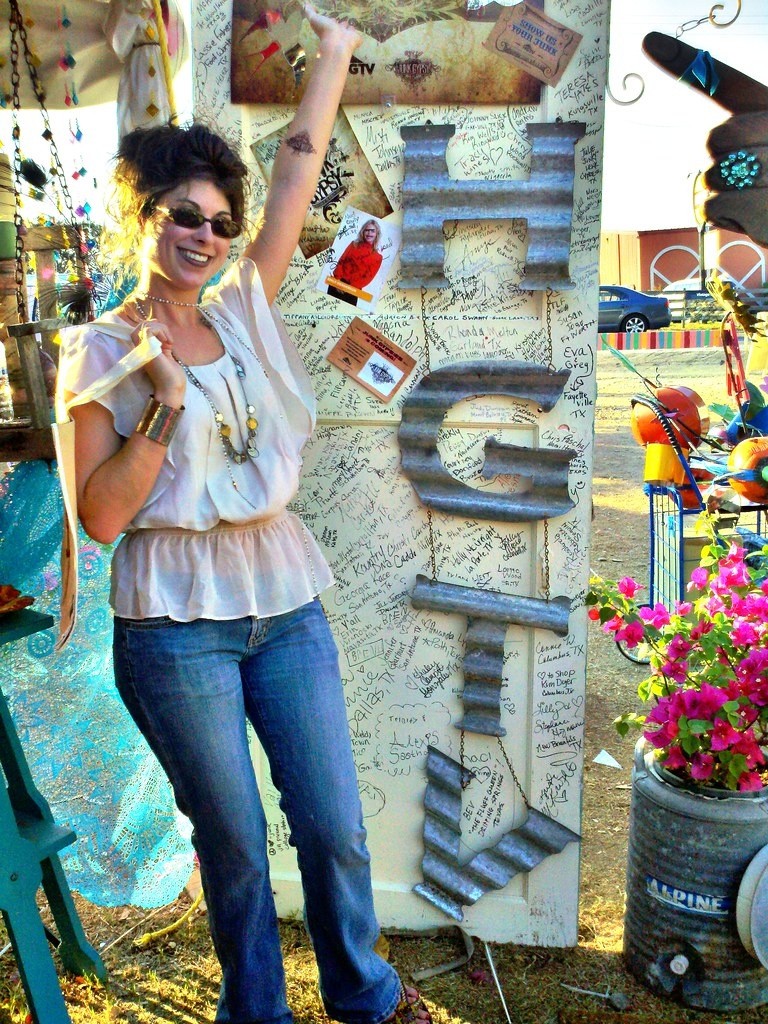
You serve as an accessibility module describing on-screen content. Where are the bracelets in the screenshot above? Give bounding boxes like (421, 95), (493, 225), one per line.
(134, 394), (186, 447)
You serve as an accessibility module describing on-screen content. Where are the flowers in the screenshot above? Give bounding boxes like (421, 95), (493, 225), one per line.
(588, 501), (768, 793)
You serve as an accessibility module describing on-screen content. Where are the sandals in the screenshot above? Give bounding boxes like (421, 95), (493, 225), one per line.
(380, 981), (433, 1024)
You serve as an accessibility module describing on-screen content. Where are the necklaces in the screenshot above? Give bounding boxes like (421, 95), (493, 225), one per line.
(132, 286), (267, 509)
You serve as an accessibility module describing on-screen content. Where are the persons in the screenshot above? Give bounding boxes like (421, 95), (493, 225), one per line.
(327, 219), (383, 306)
(54, 3), (434, 1024)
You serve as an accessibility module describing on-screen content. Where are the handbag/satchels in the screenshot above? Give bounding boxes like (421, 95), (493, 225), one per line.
(50, 323), (161, 652)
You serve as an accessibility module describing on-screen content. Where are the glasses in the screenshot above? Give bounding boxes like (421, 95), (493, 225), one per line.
(150, 204), (242, 239)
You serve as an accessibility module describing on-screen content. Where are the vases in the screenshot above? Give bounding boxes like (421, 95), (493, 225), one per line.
(622, 736), (768, 1013)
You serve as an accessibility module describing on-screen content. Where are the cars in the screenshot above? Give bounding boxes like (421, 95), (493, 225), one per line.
(657, 276), (736, 323)
(597, 285), (672, 333)
